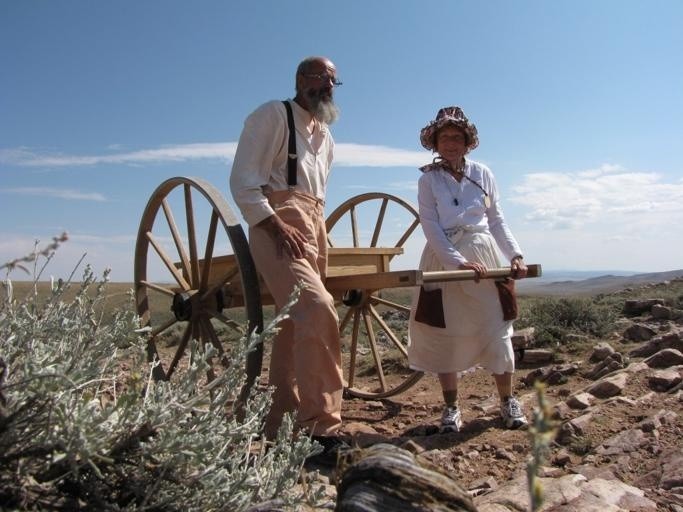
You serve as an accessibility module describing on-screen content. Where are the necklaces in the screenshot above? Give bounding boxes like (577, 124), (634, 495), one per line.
(438, 162), (464, 208)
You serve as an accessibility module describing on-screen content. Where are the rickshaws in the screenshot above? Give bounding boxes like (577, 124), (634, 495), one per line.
(132, 173), (542, 426)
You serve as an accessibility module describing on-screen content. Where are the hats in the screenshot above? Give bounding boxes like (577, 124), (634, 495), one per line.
(420, 106), (480, 154)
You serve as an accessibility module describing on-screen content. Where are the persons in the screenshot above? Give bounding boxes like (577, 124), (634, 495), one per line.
(406, 107), (530, 429)
(227, 58), (347, 486)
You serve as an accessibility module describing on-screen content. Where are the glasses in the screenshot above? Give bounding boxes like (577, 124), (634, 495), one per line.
(305, 73), (340, 88)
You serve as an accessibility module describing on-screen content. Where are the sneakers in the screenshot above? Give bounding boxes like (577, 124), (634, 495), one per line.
(499, 397), (528, 429)
(296, 432), (354, 466)
(440, 405), (462, 434)
